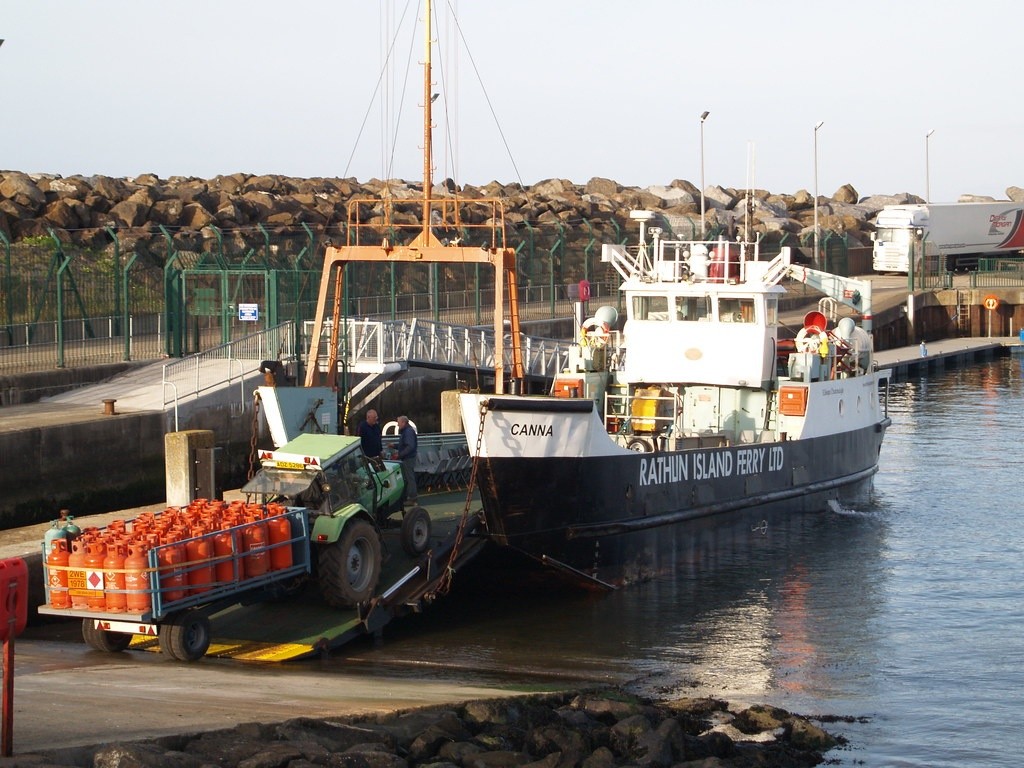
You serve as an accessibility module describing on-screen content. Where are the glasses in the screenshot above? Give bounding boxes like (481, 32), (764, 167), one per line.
(369, 416), (378, 420)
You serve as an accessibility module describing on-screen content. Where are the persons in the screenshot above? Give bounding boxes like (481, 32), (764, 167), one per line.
(388, 416), (418, 506)
(357, 409), (384, 460)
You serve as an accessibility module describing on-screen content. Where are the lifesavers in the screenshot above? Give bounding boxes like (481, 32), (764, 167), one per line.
(794, 325), (823, 354)
(627, 439), (649, 453)
(580, 318), (610, 348)
(381, 419), (418, 435)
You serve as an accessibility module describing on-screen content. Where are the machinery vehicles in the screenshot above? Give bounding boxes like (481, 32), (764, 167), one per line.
(34, 431), (432, 664)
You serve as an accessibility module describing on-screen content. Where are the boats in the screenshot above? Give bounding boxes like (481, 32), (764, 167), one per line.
(252, 210), (893, 588)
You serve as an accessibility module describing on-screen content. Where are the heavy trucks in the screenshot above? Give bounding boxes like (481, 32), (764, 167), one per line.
(870, 201), (1024, 276)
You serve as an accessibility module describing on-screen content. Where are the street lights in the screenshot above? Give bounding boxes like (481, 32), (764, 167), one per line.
(700, 110), (712, 242)
(813, 121), (824, 269)
(925, 129), (936, 203)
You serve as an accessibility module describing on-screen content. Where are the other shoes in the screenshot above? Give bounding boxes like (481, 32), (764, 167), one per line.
(403, 499), (418, 506)
